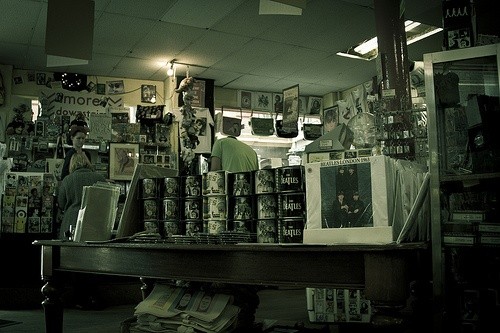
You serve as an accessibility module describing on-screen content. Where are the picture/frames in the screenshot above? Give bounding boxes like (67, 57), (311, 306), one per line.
(141, 84), (157, 103)
(322, 105), (339, 137)
(109, 142), (141, 181)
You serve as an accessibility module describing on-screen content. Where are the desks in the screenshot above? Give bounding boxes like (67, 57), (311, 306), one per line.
(32, 238), (430, 333)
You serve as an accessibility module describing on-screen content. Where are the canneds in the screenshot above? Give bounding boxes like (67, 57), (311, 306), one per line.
(141, 165), (307, 244)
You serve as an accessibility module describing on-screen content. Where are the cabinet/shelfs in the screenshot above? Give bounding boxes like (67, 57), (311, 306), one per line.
(423, 41), (500, 333)
(139, 117), (176, 170)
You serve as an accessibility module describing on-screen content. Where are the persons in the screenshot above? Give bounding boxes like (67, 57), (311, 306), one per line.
(211, 135), (259, 172)
(61, 125), (92, 180)
(59, 152), (107, 238)
(334, 165), (365, 227)
